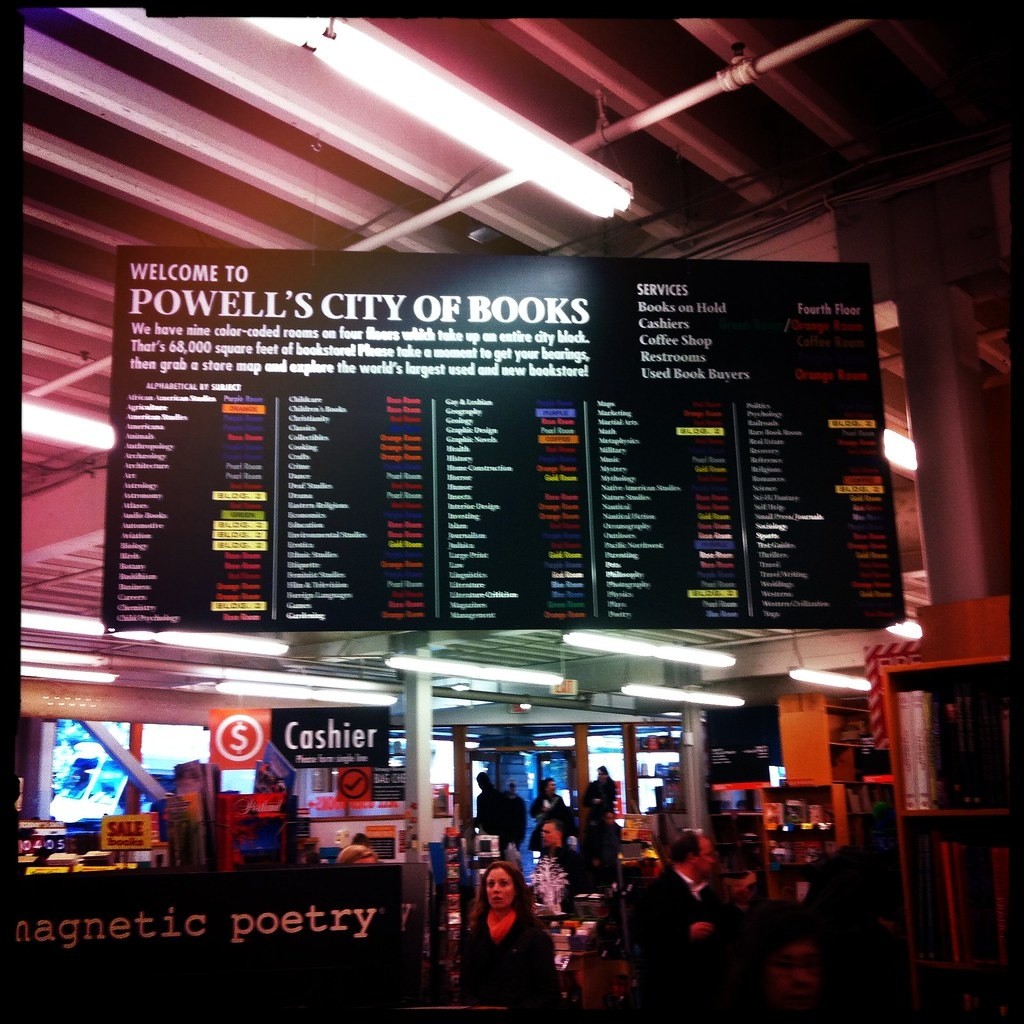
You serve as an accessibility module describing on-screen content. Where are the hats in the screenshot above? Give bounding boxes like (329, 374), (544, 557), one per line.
(337, 845), (372, 864)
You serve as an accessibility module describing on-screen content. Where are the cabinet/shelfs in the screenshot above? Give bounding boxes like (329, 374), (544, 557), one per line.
(759, 782), (849, 902)
(827, 703), (893, 847)
(713, 812), (765, 876)
(633, 721), (688, 814)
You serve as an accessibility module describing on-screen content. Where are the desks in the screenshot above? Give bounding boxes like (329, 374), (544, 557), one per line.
(555, 951), (628, 1008)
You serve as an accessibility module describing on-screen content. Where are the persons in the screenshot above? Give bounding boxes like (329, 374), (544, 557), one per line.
(459, 861), (564, 1004)
(626, 830), (822, 1024)
(476, 766), (620, 896)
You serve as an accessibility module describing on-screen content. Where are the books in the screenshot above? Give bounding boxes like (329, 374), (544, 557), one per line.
(894, 692), (1024, 963)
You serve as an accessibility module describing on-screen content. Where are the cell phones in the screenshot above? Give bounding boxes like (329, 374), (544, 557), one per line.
(49, 749), (129, 823)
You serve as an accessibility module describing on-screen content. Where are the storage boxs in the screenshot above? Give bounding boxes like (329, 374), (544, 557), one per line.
(841, 730), (859, 740)
(660, 736), (670, 750)
(804, 841), (823, 862)
(640, 737), (649, 750)
(825, 841), (835, 853)
(786, 800), (807, 823)
(797, 881), (814, 902)
(648, 735), (659, 750)
(470, 833), (609, 952)
(794, 841), (805, 864)
(764, 802), (785, 824)
(807, 805), (831, 822)
(769, 840), (795, 863)
(848, 720), (867, 735)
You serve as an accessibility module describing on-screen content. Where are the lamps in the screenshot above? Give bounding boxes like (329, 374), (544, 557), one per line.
(620, 657), (746, 709)
(19, 607), (398, 706)
(786, 629), (874, 693)
(382, 630), (566, 685)
(563, 628), (737, 668)
(255, 15), (636, 220)
